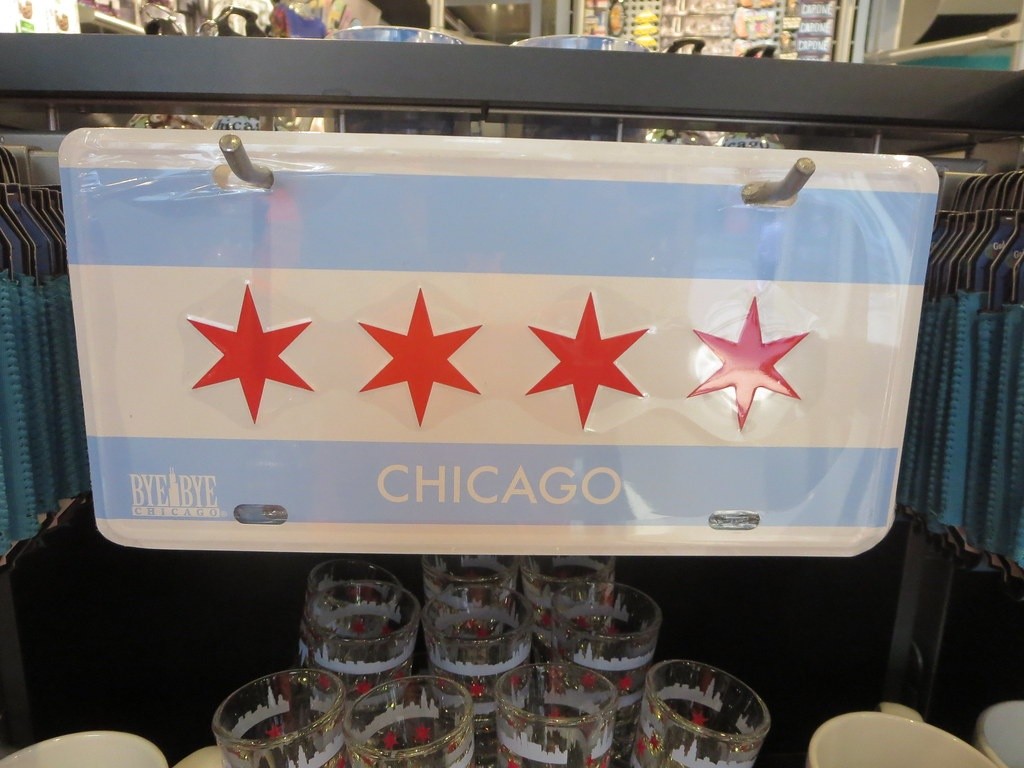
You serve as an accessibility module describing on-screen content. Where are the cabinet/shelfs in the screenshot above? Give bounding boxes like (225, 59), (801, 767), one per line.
(572, 0), (856, 63)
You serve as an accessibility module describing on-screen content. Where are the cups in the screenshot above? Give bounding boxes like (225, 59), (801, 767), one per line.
(210, 556), (772, 768)
(971, 699), (1024, 768)
(804, 702), (996, 768)
(509, 35), (649, 143)
(0, 730), (169, 768)
(323, 25), (473, 137)
